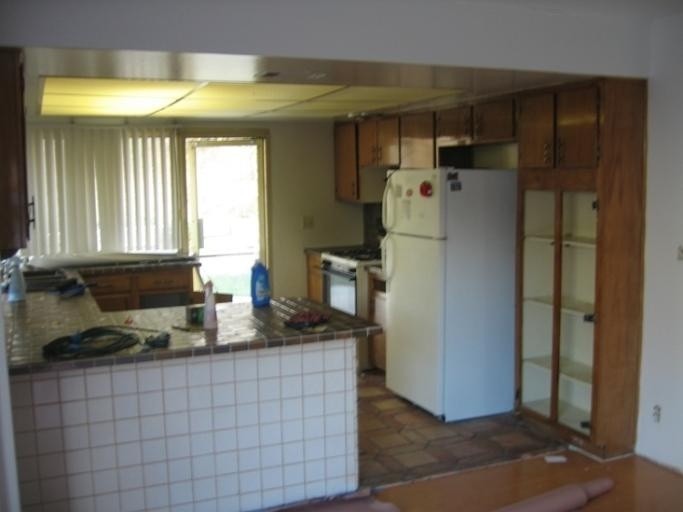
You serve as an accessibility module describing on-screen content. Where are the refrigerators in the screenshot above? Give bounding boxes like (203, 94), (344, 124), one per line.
(380, 166), (522, 426)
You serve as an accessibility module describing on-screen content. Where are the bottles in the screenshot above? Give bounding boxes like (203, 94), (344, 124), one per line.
(6, 263), (27, 302)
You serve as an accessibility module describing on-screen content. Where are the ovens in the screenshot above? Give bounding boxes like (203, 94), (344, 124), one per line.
(317, 268), (370, 373)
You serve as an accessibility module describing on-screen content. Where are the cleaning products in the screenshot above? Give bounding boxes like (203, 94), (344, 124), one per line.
(6, 257), (27, 302)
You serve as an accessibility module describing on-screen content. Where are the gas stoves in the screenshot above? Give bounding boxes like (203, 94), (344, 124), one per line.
(320, 243), (381, 271)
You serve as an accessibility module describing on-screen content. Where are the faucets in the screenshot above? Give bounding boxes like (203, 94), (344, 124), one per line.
(2, 258), (29, 283)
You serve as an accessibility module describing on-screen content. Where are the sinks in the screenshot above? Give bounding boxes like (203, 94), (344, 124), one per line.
(8, 268), (65, 279)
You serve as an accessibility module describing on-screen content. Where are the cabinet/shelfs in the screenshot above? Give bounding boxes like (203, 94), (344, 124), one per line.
(370, 278), (385, 372)
(306, 253), (322, 302)
(433, 96), (515, 145)
(0, 47), (36, 261)
(84, 267), (232, 311)
(356, 117), (399, 202)
(332, 121), (358, 202)
(399, 112), (434, 168)
